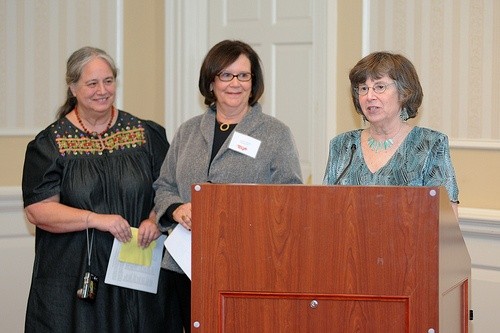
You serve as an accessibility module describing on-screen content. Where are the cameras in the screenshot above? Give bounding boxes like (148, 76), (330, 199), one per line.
(77, 272), (99, 303)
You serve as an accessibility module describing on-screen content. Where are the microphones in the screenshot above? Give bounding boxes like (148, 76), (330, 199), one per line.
(334, 144), (356, 185)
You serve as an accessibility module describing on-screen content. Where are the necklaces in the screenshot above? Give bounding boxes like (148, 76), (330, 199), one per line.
(365, 123), (407, 153)
(213, 106), (251, 131)
(73, 103), (115, 137)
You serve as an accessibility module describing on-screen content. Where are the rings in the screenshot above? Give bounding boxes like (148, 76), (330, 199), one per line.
(181, 214), (187, 220)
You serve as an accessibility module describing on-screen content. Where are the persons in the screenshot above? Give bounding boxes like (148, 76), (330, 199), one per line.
(322, 51), (459, 223)
(151, 39), (304, 333)
(21, 45), (171, 333)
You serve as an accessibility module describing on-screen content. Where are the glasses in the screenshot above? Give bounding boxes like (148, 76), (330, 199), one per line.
(214, 71), (254, 82)
(354, 81), (397, 96)
